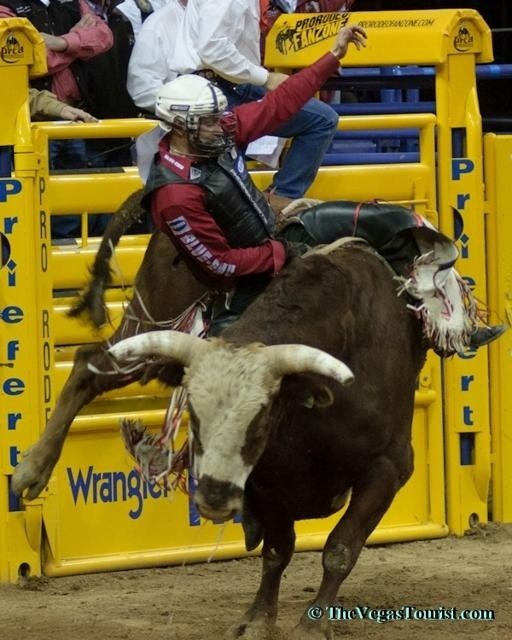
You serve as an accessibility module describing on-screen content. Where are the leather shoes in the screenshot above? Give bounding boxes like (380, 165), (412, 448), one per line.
(429, 319), (510, 360)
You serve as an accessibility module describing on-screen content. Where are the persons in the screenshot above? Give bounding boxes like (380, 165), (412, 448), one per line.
(260, 0), (350, 102)
(168, 1), (339, 209)
(0, 0), (192, 263)
(121, 22), (509, 483)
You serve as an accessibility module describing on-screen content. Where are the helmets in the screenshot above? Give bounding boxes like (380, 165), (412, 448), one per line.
(153, 72), (229, 135)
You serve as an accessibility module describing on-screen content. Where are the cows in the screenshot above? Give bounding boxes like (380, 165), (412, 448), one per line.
(8, 177), (429, 639)
